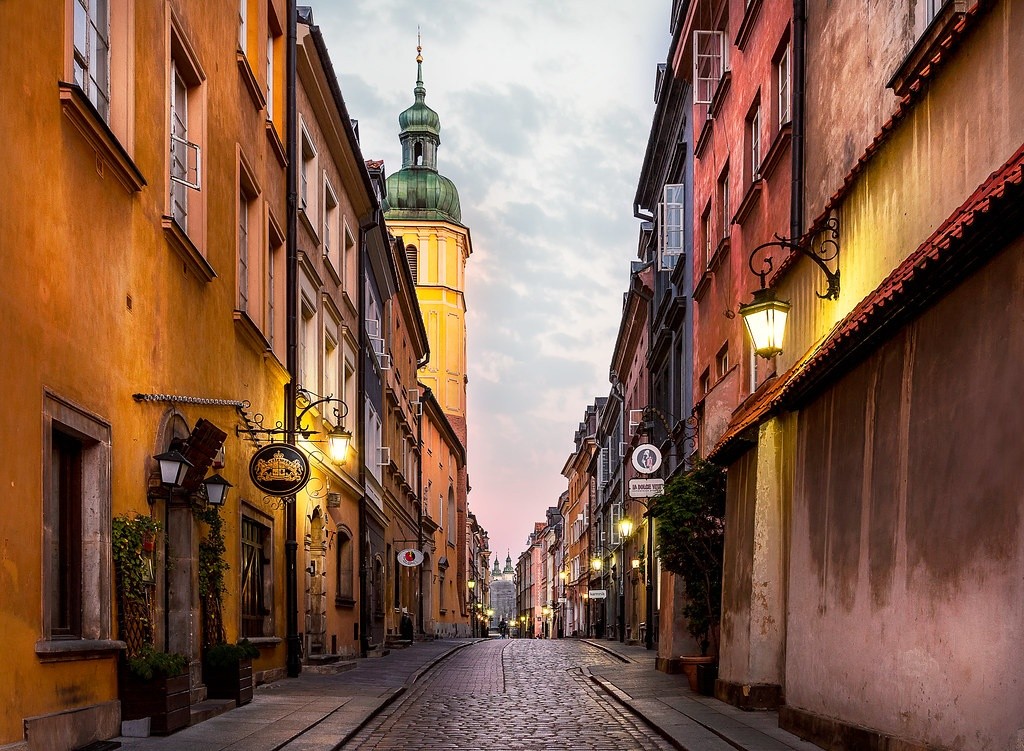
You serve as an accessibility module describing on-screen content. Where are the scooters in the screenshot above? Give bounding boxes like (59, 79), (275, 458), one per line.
(498, 623), (507, 638)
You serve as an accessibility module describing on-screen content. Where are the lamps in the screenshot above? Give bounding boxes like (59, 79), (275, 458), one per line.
(631, 559), (643, 570)
(593, 546), (612, 572)
(235, 383), (353, 467)
(616, 498), (650, 538)
(148, 447), (196, 506)
(200, 474), (233, 518)
(738, 201), (840, 360)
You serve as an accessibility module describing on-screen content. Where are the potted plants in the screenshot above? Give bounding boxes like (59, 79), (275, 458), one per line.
(202, 639), (260, 706)
(653, 461), (727, 691)
(126, 644), (192, 735)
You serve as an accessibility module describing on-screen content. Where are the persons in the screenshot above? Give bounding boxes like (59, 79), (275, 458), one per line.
(498, 618), (506, 638)
